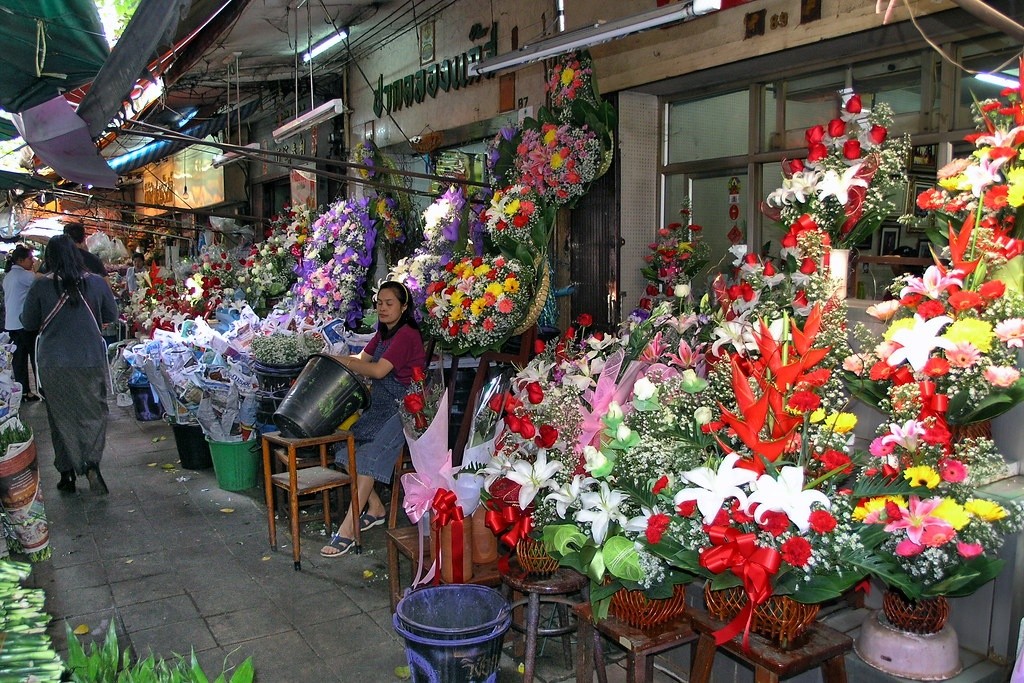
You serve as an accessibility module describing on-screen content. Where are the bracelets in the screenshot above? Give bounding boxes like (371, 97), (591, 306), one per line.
(347, 359), (355, 366)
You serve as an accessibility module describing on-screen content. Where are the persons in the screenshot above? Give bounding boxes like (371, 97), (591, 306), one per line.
(125, 254), (148, 293)
(65, 224), (111, 330)
(320, 282), (424, 558)
(3, 244), (43, 401)
(19, 236), (118, 496)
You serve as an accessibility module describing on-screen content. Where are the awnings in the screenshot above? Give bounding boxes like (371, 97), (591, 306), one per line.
(0, 2), (493, 198)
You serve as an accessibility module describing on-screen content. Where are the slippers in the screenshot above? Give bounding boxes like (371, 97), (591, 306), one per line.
(359, 510), (386, 531)
(320, 531), (357, 557)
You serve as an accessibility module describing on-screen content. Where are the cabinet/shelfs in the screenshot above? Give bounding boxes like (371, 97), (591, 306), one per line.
(262, 430), (363, 570)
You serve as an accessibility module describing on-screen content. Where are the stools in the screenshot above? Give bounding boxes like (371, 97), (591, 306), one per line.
(385, 438), (415, 529)
(690, 609), (853, 683)
(415, 553), (505, 589)
(573, 600), (698, 683)
(496, 556), (591, 683)
(387, 527), (430, 615)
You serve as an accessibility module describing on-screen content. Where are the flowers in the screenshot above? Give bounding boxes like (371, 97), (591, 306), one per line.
(236, 56), (1024, 597)
(136, 249), (232, 331)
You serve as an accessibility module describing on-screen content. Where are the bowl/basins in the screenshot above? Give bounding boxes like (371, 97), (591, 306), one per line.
(851, 610), (962, 681)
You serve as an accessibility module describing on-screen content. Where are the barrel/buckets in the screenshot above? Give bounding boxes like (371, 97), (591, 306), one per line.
(204, 432), (259, 491)
(129, 377), (165, 421)
(272, 353), (372, 438)
(392, 584), (512, 683)
(169, 421), (212, 469)
(249, 389), (290, 478)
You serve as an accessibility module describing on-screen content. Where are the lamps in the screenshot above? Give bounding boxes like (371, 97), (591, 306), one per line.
(273, 0), (343, 145)
(302, 26), (350, 64)
(214, 53), (259, 166)
(477, 0), (721, 79)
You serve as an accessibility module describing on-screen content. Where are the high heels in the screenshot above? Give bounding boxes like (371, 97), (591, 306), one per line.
(57, 469), (76, 495)
(21, 393), (40, 402)
(84, 463), (109, 495)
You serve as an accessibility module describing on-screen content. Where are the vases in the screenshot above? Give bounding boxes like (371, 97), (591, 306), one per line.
(706, 575), (821, 643)
(583, 118), (614, 179)
(514, 520), (562, 576)
(511, 251), (551, 338)
(882, 587), (951, 634)
(594, 577), (687, 631)
(825, 248), (853, 301)
(947, 417), (994, 457)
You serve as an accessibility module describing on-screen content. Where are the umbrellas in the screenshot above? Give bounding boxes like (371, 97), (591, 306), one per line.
(12, 95), (123, 194)
